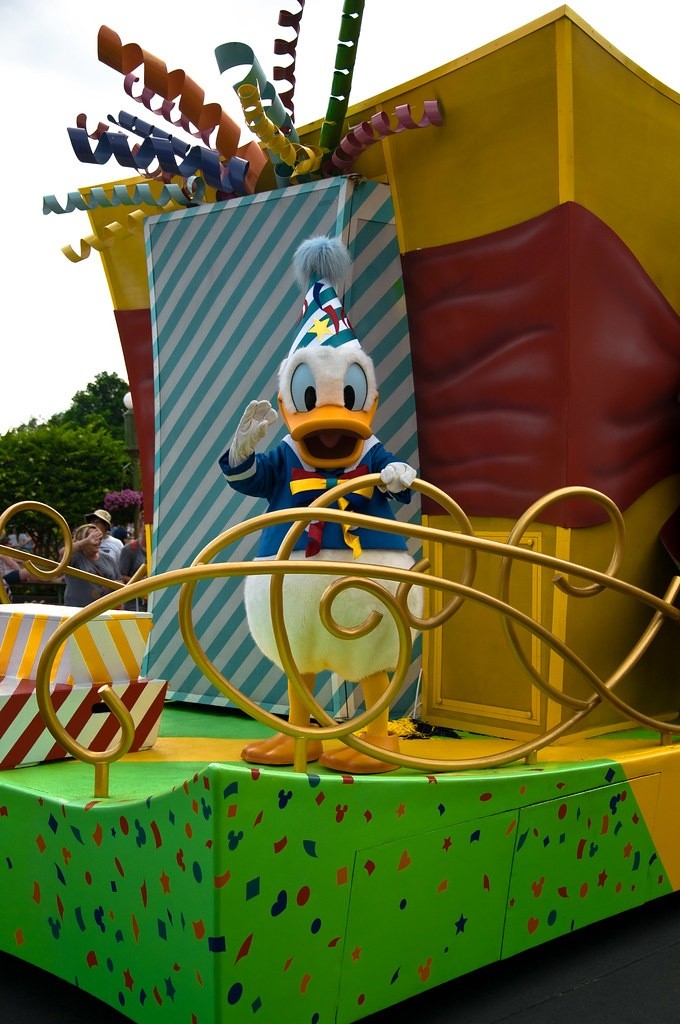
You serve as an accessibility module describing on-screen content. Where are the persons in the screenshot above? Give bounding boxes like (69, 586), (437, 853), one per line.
(120, 510), (147, 612)
(58, 524), (122, 608)
(9, 527), (36, 553)
(84, 508), (124, 567)
(0, 538), (48, 584)
(109, 520), (135, 545)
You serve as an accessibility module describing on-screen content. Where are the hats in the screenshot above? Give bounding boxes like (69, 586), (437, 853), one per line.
(84, 509), (112, 532)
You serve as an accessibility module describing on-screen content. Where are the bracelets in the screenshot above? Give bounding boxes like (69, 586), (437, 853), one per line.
(76, 540), (82, 551)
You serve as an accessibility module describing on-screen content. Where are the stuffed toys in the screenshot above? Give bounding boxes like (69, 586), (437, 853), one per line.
(217, 234), (416, 774)
